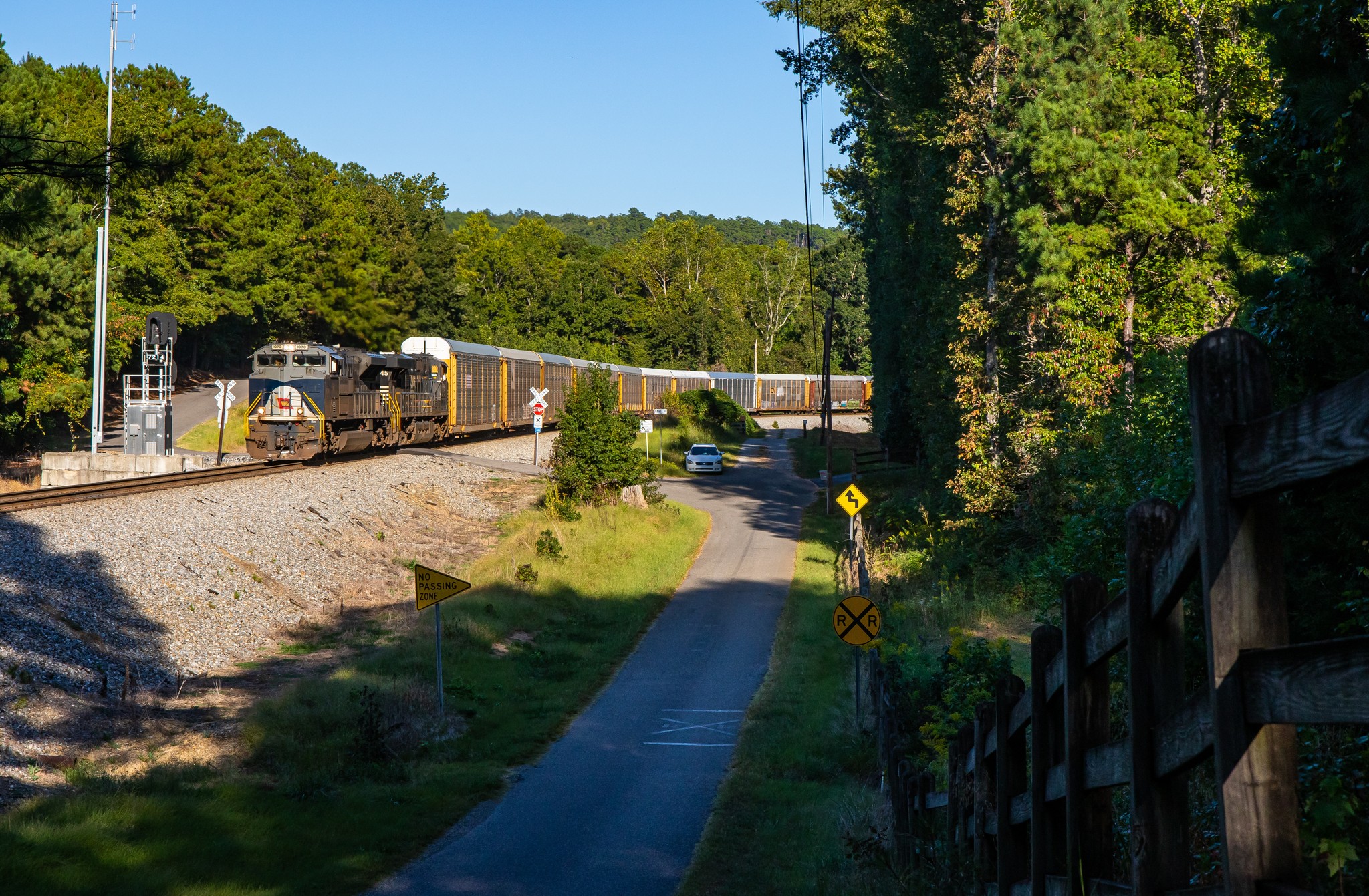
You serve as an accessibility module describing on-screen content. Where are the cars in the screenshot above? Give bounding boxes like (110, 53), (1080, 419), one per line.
(683, 444), (725, 473)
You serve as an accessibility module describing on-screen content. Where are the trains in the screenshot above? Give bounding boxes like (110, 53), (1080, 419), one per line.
(243, 336), (873, 466)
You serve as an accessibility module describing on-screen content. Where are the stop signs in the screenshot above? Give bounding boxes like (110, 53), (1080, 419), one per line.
(533, 403), (545, 416)
